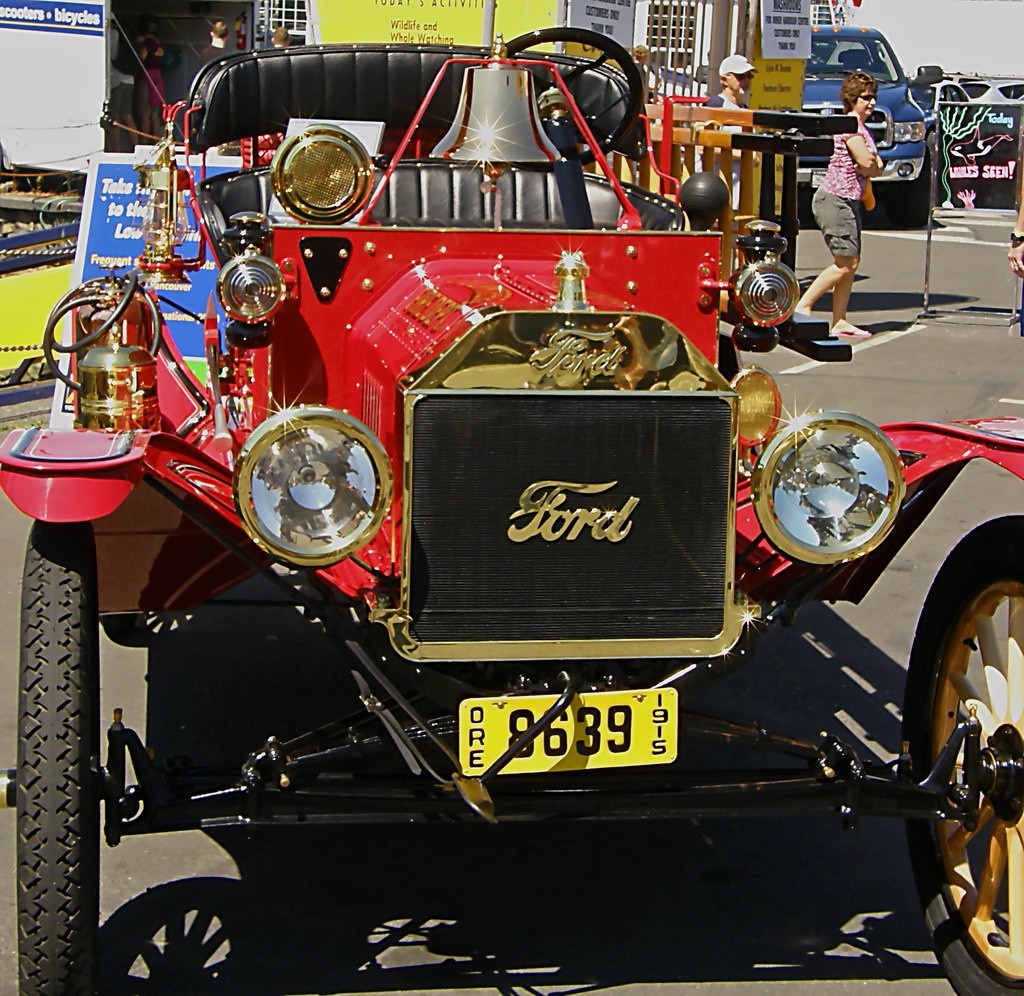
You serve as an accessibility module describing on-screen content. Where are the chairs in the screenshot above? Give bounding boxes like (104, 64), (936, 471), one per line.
(839, 48), (873, 69)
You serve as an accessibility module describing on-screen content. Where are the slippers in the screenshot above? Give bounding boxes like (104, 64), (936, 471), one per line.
(830, 328), (872, 340)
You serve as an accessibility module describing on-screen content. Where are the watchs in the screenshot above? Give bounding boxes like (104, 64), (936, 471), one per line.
(1011, 228), (1024, 243)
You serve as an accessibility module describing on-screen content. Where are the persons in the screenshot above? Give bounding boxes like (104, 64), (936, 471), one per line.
(633, 44), (663, 104)
(109, 22), (165, 151)
(1007, 196), (1024, 336)
(199, 21), (234, 71)
(271, 27), (291, 47)
(796, 72), (883, 339)
(694, 55), (758, 209)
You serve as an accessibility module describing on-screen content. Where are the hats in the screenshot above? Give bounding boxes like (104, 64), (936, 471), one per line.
(718, 55), (758, 77)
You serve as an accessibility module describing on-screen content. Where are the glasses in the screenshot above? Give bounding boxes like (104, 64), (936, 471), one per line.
(859, 94), (878, 100)
(736, 73), (754, 80)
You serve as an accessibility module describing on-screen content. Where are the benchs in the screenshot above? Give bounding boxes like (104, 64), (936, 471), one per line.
(186, 42), (686, 270)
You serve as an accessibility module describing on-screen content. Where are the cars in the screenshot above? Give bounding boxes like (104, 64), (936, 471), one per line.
(0, 30), (1023, 993)
(759, 25), (946, 227)
